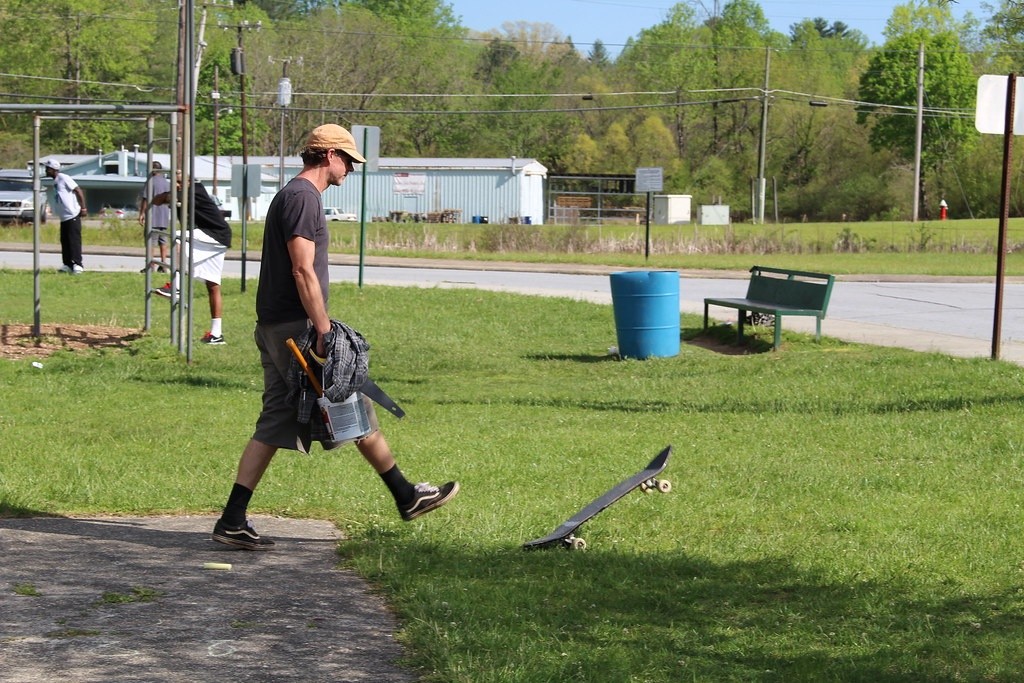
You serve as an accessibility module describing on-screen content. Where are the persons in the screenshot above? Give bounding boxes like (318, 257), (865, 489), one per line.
(139, 161), (170, 273)
(212, 124), (460, 547)
(153, 171), (231, 345)
(43, 159), (87, 272)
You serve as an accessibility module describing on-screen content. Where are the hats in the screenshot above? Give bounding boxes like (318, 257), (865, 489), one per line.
(42, 159), (60, 170)
(305, 124), (367, 163)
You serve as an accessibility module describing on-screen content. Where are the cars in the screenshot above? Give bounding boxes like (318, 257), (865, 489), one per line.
(100, 202), (141, 221)
(323, 207), (358, 223)
(0, 168), (50, 225)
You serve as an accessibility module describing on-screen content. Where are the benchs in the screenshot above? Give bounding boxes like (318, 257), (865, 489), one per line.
(704, 266), (834, 351)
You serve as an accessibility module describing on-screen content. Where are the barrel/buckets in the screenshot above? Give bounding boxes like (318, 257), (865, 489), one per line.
(317, 365), (372, 443)
(608, 270), (680, 360)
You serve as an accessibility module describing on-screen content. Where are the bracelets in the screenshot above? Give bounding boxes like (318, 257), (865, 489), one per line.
(81, 209), (87, 211)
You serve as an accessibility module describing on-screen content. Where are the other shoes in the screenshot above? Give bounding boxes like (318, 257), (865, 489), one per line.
(157, 267), (165, 274)
(140, 268), (146, 273)
(59, 265), (71, 272)
(73, 263), (83, 273)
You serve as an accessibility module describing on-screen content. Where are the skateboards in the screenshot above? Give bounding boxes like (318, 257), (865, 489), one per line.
(524, 444), (673, 549)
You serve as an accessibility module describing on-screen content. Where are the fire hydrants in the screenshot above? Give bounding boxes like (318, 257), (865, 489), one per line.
(939, 199), (949, 220)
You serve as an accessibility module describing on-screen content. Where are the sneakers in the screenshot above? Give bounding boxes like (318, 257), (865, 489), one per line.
(156, 283), (180, 297)
(200, 332), (224, 346)
(213, 519), (275, 550)
(398, 481), (459, 520)
